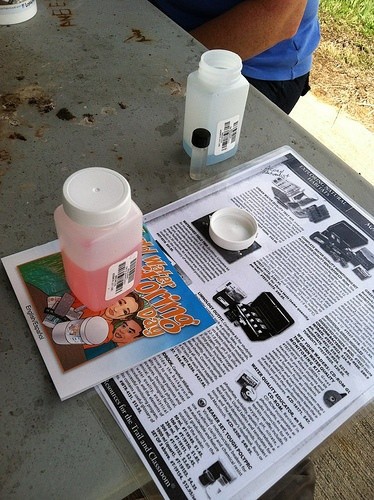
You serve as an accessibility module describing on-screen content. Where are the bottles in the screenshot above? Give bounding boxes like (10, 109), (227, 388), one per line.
(182, 49), (250, 166)
(190, 128), (211, 180)
(53, 166), (144, 311)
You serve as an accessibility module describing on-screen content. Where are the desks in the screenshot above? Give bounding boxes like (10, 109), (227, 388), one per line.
(0, 0), (374, 500)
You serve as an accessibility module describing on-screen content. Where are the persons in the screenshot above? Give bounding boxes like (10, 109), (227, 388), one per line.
(148, 0), (321, 117)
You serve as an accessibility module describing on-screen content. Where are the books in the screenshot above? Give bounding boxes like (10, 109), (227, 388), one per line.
(2, 220), (217, 401)
